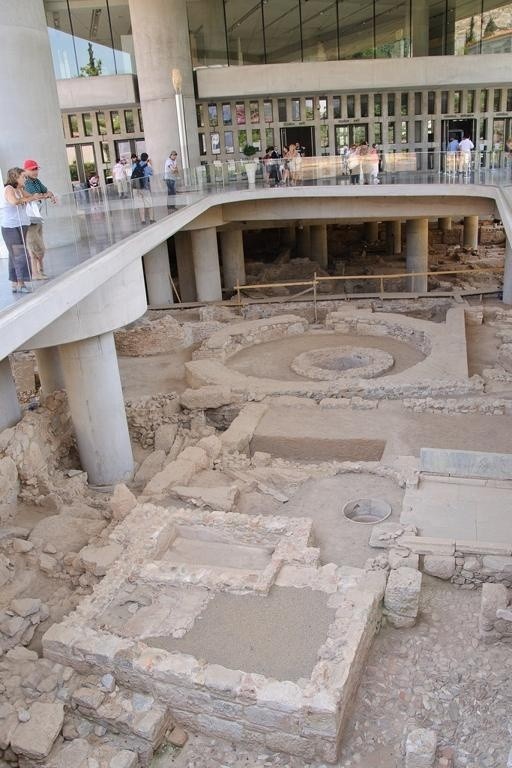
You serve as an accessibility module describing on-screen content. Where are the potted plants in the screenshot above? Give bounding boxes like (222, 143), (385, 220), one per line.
(243, 144), (258, 183)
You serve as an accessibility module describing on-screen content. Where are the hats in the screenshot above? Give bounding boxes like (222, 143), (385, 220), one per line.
(24, 160), (40, 171)
(170, 151), (178, 155)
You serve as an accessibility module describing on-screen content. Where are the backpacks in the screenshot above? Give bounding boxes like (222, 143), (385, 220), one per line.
(132, 162), (148, 189)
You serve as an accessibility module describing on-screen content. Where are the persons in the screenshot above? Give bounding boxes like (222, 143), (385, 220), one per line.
(129, 152), (157, 224)
(89, 175), (100, 193)
(163, 150), (180, 215)
(445, 134), (475, 174)
(261, 141), (306, 184)
(338, 139), (385, 184)
(113, 159), (128, 199)
(131, 154), (141, 171)
(1, 166), (41, 293)
(19, 159), (57, 280)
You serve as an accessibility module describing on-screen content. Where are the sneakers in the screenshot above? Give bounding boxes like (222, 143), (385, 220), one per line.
(10, 286), (29, 293)
(141, 220), (155, 225)
(32, 273), (48, 280)
